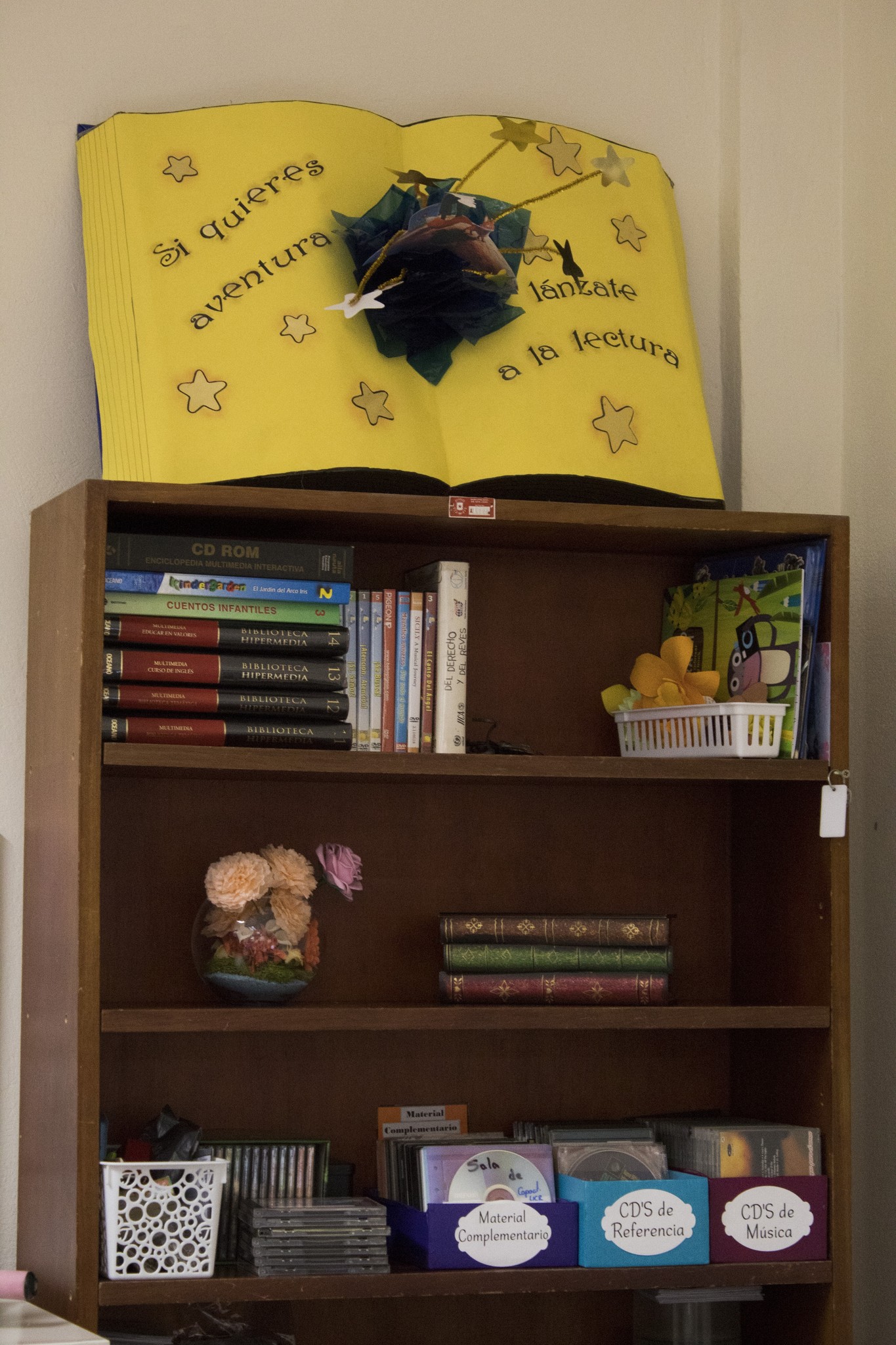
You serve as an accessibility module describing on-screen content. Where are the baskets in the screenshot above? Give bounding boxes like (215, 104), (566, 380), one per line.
(100, 1156), (229, 1278)
(613, 703), (790, 758)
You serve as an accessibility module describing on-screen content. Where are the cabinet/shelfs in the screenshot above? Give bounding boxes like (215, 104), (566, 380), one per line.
(16, 477), (864, 1345)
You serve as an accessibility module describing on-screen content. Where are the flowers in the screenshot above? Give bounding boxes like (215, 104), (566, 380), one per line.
(196, 835), (363, 945)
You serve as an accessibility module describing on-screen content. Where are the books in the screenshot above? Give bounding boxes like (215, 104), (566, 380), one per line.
(426, 902), (680, 1007)
(72, 99), (731, 510)
(99, 518), (847, 761)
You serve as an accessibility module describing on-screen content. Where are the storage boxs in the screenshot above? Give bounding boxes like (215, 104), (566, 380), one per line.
(372, 1197), (577, 1274)
(555, 1168), (710, 1267)
(691, 1168), (828, 1263)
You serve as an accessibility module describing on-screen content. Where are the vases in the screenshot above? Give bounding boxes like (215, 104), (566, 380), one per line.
(192, 894), (321, 1006)
